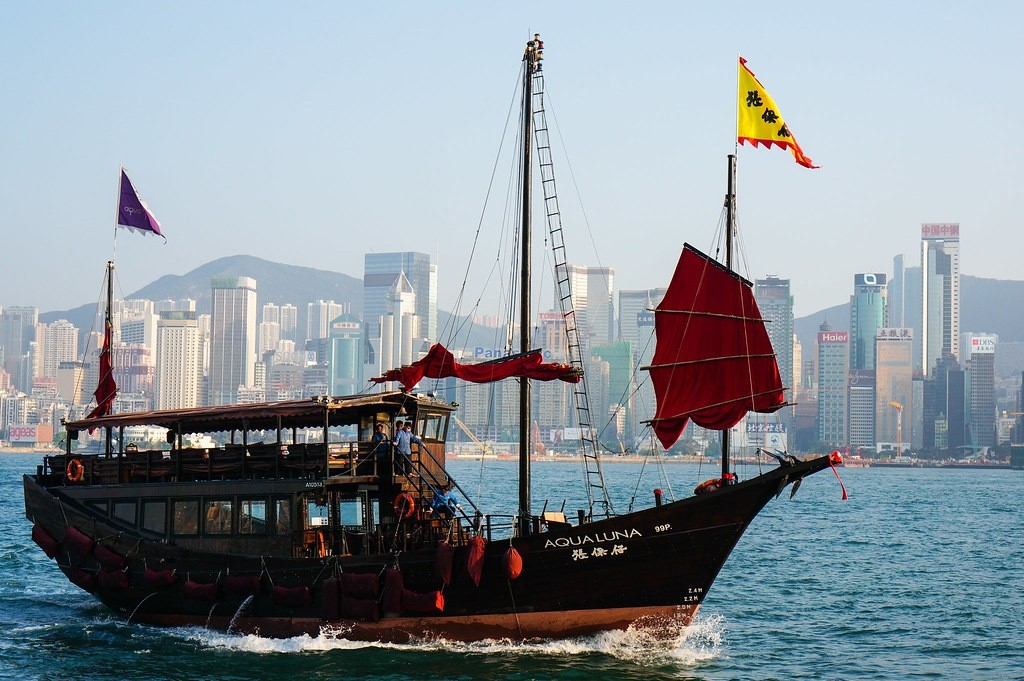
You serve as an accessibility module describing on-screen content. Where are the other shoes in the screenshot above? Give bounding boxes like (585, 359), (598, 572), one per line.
(407, 473), (420, 478)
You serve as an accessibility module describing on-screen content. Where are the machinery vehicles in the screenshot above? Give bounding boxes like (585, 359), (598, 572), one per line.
(887, 400), (903, 463)
(1002, 410), (1024, 418)
(452, 414), (497, 456)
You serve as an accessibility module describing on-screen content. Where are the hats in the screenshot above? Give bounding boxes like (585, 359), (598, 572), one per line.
(442, 484), (451, 491)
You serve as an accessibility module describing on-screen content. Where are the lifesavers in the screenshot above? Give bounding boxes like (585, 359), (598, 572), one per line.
(394, 493), (415, 519)
(694, 478), (721, 494)
(67, 459), (82, 482)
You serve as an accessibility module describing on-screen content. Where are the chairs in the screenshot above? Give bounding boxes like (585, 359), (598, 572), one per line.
(50, 443), (330, 482)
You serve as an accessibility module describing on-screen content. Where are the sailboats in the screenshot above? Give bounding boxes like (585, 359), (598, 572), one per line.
(22, 26), (842, 659)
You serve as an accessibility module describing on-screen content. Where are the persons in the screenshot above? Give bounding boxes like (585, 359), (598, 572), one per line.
(371, 420), (426, 478)
(431, 485), (464, 524)
